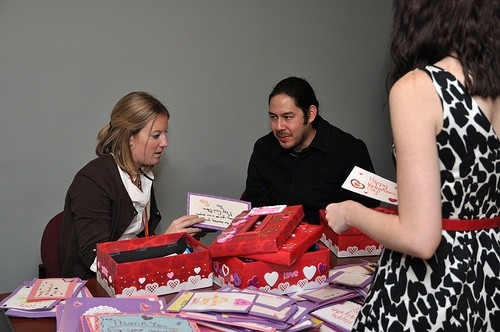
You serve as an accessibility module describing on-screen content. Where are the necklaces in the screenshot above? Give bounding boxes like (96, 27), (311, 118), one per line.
(130, 174), (141, 188)
(448, 54), (459, 60)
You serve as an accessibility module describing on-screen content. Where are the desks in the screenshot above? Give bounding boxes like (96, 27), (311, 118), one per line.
(0, 250), (379, 332)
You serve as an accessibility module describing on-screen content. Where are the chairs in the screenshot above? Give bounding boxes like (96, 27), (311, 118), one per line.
(37, 210), (64, 278)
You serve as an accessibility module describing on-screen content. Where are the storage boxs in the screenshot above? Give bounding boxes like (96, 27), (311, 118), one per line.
(95, 205), (400, 300)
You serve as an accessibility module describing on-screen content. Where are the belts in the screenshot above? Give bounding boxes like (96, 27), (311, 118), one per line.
(441, 215), (500, 231)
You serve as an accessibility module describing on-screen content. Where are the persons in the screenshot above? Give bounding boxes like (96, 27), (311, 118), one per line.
(60, 91), (218, 280)
(325, 0), (500, 332)
(241, 77), (380, 225)
